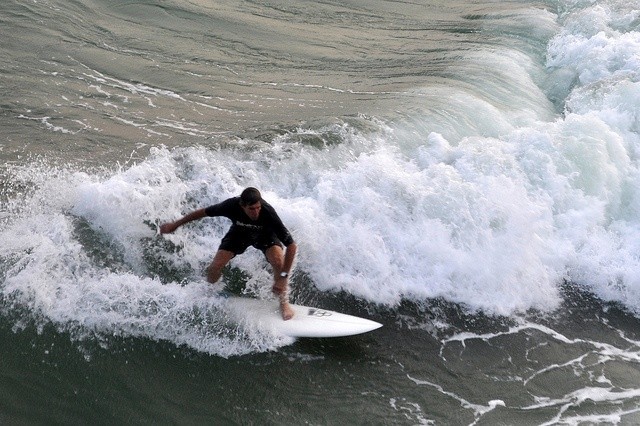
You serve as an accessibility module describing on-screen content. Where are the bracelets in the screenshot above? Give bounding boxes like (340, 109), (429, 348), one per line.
(279, 271), (289, 279)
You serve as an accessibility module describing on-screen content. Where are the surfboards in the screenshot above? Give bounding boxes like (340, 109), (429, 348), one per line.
(231, 296), (383, 339)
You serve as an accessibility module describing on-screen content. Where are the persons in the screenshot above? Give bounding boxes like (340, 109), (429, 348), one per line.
(159, 186), (297, 321)
